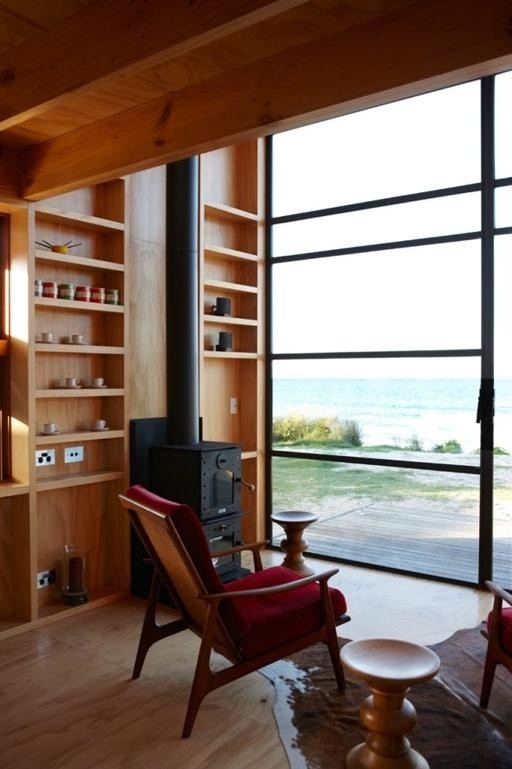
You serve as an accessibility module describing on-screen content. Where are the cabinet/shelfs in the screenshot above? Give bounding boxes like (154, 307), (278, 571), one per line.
(0, 174), (131, 641)
(198, 136), (267, 549)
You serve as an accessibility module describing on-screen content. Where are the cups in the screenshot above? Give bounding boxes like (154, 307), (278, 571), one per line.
(94, 378), (105, 386)
(96, 419), (108, 428)
(41, 332), (53, 341)
(65, 377), (80, 386)
(72, 334), (86, 343)
(43, 423), (58, 433)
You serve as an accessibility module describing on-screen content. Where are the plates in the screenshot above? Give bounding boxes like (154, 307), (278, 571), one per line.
(91, 426), (110, 431)
(69, 342), (88, 345)
(88, 384), (109, 388)
(63, 385), (84, 389)
(39, 430), (61, 435)
(36, 341), (57, 344)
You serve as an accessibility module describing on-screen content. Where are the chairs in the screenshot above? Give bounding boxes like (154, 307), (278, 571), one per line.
(481, 580), (512, 708)
(118, 485), (351, 738)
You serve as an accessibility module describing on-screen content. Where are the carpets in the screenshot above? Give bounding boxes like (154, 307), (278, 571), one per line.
(257, 621), (512, 769)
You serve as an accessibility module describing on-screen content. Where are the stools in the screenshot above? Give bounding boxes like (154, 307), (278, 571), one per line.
(339, 639), (440, 769)
(269, 511), (319, 577)
